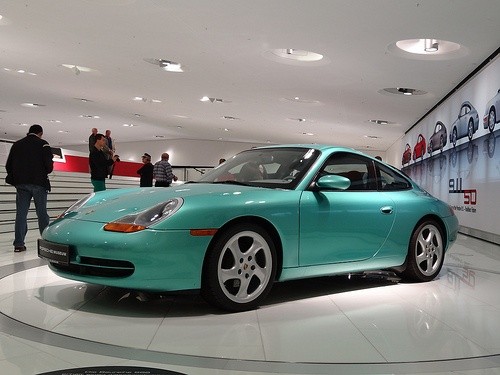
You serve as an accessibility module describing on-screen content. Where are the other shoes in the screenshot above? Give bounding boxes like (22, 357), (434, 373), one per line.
(14, 245), (26, 252)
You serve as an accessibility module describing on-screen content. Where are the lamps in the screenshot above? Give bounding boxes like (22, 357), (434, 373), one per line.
(424, 38), (439, 52)
(402, 88), (415, 95)
(378, 121), (389, 125)
(287, 48), (293, 54)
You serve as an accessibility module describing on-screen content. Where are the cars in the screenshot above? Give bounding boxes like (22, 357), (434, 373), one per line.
(447, 101), (480, 147)
(402, 143), (412, 167)
(427, 121), (447, 155)
(412, 133), (426, 162)
(482, 88), (500, 133)
(37, 143), (459, 315)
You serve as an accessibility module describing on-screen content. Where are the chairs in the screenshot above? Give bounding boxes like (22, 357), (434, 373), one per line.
(238, 161), (263, 182)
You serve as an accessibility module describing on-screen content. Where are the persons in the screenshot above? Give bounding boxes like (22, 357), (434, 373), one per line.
(5, 125), (54, 253)
(218, 159), (235, 181)
(240, 156), (266, 180)
(153, 153), (178, 187)
(88, 128), (98, 151)
(137, 153), (154, 188)
(105, 130), (116, 153)
(88, 134), (117, 192)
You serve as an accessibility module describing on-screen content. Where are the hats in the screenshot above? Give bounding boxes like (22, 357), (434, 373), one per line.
(142, 153), (151, 158)
